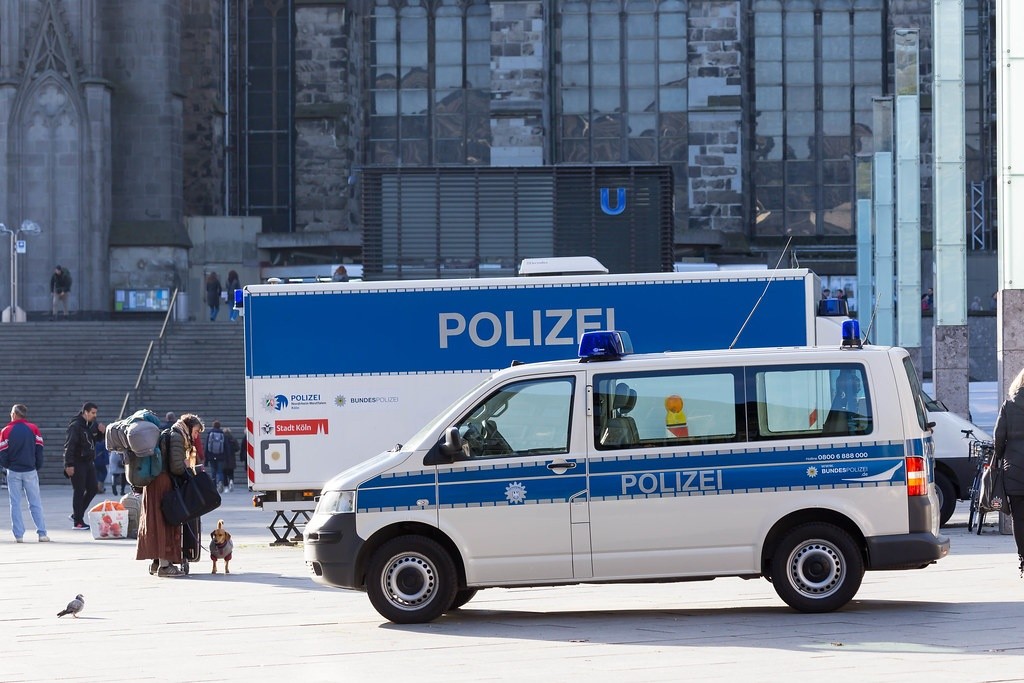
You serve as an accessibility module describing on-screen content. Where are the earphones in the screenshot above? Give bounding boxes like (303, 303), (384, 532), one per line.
(85, 412), (87, 414)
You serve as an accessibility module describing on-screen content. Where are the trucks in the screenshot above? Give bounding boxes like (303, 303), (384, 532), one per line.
(228, 252), (992, 527)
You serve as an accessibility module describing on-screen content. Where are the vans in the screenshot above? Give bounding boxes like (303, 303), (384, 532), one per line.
(303, 326), (952, 624)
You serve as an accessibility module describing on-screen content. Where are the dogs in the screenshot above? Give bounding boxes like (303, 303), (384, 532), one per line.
(209, 519), (233, 574)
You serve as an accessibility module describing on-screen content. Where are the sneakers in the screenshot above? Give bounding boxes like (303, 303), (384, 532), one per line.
(156, 563), (185, 577)
(69, 513), (75, 521)
(72, 520), (89, 529)
(148, 560), (159, 574)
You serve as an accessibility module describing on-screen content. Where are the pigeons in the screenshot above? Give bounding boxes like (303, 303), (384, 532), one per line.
(56, 594), (85, 618)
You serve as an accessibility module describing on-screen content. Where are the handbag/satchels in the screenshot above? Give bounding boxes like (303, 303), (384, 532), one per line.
(978, 455), (1011, 516)
(161, 468), (221, 527)
(87, 499), (128, 540)
(119, 459), (124, 468)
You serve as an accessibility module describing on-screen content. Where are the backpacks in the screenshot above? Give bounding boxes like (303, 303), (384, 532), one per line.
(121, 488), (143, 538)
(106, 410), (169, 484)
(207, 431), (224, 454)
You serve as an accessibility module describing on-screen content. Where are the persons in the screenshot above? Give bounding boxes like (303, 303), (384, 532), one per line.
(0, 404), (51, 543)
(333, 266), (349, 282)
(970, 292), (997, 311)
(135, 414), (206, 577)
(48, 265), (72, 322)
(94, 412), (247, 496)
(63, 402), (106, 530)
(921, 287), (933, 316)
(225, 270), (241, 322)
(822, 289), (850, 316)
(206, 271), (222, 321)
(994, 369), (1024, 578)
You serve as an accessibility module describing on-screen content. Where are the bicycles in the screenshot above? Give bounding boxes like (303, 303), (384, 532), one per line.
(958, 429), (1001, 535)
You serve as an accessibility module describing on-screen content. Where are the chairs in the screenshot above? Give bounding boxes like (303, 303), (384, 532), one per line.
(620, 389), (640, 444)
(599, 383), (634, 444)
(821, 395), (848, 433)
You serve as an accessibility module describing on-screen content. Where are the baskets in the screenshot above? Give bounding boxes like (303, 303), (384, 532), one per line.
(967, 440), (994, 465)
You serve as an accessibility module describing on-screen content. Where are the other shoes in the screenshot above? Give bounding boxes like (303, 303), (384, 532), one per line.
(39, 534), (50, 542)
(217, 480), (224, 494)
(16, 536), (24, 543)
(112, 485), (118, 496)
(121, 491), (127, 495)
(229, 479), (234, 491)
